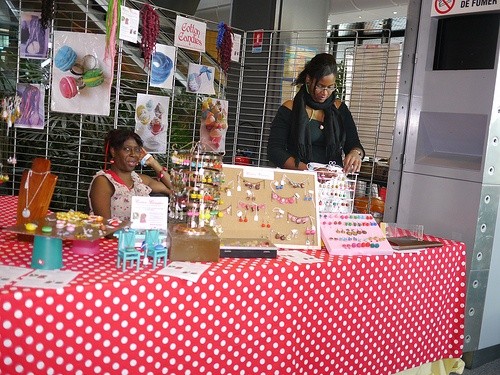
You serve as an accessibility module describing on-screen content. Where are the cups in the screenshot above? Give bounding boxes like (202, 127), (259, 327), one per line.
(71, 240), (100, 255)
(30, 235), (63, 270)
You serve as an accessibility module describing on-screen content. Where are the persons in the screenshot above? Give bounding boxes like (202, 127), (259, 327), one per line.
(267, 53), (366, 174)
(88, 129), (172, 239)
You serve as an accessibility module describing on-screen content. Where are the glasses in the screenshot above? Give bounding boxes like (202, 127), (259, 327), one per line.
(313, 82), (337, 91)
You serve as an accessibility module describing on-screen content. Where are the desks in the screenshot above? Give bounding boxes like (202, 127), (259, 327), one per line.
(0, 195), (466, 375)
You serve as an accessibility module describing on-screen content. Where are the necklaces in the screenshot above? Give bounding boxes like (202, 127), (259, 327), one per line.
(22, 169), (50, 219)
(308, 108), (328, 129)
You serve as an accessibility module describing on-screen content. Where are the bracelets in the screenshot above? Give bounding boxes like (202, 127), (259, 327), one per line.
(140, 154), (152, 167)
(156, 167), (168, 182)
(351, 149), (362, 156)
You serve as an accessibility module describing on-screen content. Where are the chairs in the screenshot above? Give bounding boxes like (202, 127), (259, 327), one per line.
(117, 229), (140, 272)
(144, 229), (168, 269)
